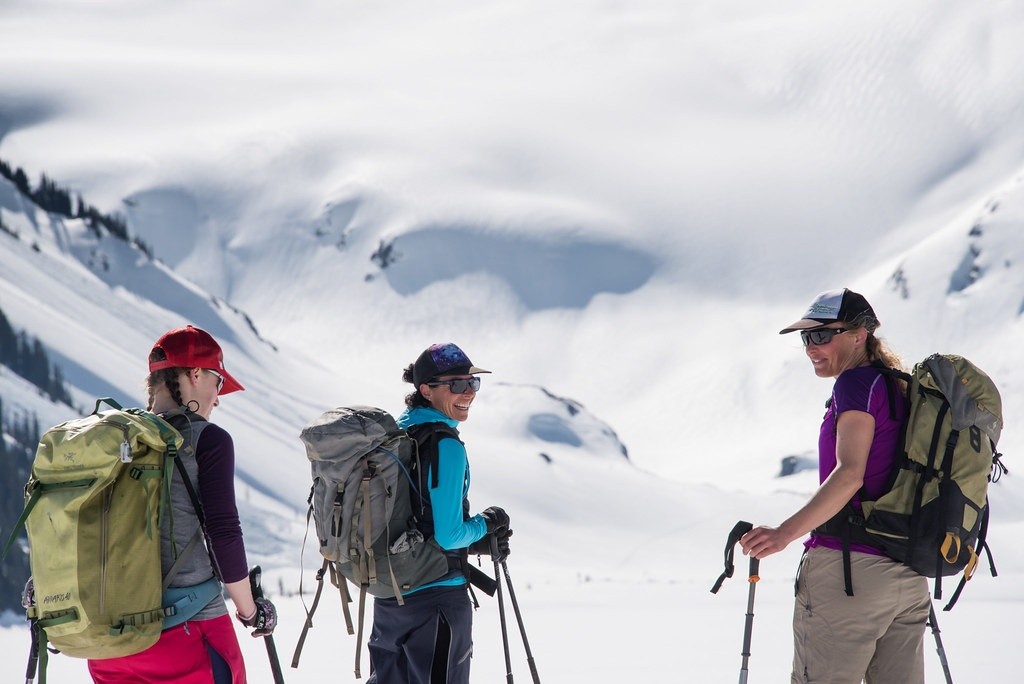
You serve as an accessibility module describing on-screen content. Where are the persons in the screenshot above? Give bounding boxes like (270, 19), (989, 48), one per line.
(85, 323), (275, 684)
(739, 289), (933, 684)
(363, 342), (519, 684)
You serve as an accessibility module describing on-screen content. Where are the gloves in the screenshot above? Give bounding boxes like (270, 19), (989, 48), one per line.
(470, 529), (512, 560)
(235, 597), (277, 638)
(482, 505), (511, 537)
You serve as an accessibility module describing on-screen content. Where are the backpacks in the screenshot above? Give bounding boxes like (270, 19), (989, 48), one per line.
(810, 352), (1008, 580)
(296, 404), (471, 599)
(22, 395), (223, 659)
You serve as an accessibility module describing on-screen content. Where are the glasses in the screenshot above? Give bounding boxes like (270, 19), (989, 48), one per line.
(800, 327), (854, 346)
(207, 369), (224, 394)
(426, 376), (481, 394)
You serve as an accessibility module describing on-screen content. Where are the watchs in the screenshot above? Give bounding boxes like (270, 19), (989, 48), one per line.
(234, 605), (258, 625)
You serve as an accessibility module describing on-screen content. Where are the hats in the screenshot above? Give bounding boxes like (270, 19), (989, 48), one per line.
(412, 342), (492, 388)
(778, 287), (878, 335)
(147, 326), (244, 396)
